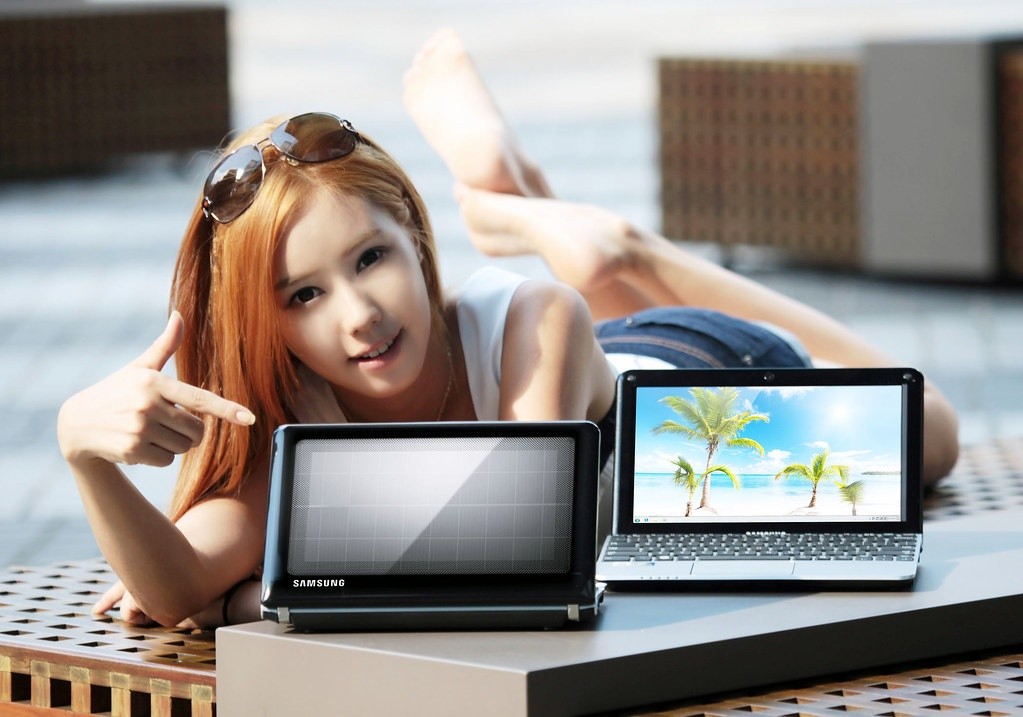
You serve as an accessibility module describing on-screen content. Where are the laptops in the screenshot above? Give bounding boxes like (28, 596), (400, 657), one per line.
(591, 364), (926, 593)
(253, 417), (607, 628)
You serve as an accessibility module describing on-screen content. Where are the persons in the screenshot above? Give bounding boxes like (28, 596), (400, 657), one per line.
(49, 27), (961, 672)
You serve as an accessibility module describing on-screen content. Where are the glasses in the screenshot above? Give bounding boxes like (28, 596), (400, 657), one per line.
(200, 111), (368, 224)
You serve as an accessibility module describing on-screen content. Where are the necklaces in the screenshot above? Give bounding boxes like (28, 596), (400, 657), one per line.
(324, 336), (453, 423)
